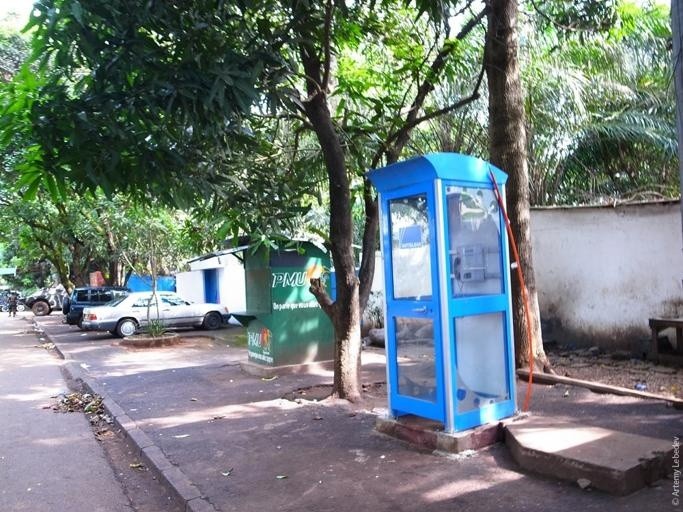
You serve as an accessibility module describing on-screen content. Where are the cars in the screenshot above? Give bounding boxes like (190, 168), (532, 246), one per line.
(81, 290), (232, 337)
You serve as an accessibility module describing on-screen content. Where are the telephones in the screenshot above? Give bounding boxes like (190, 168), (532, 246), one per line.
(454, 258), (460, 280)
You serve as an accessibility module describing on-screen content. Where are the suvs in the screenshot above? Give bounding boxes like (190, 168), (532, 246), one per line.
(20, 285), (64, 316)
(62, 287), (129, 331)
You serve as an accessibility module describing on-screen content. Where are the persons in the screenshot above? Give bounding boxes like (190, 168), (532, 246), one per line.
(5, 289), (19, 316)
